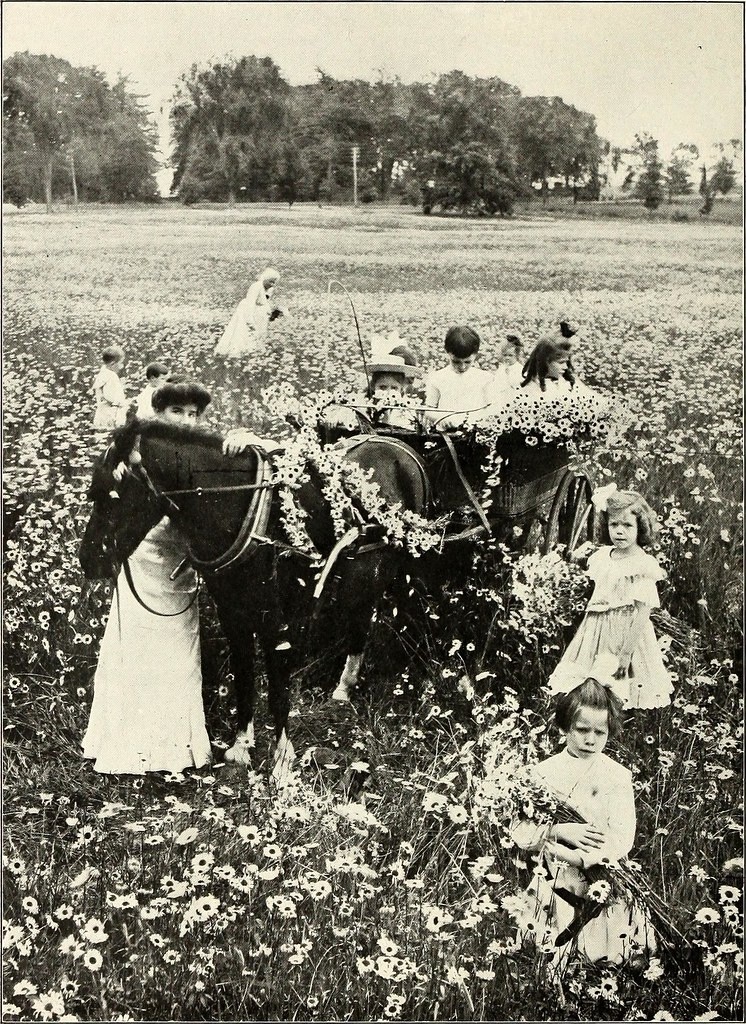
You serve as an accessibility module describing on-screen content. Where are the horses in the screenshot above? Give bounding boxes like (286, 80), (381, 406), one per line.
(79, 418), (435, 774)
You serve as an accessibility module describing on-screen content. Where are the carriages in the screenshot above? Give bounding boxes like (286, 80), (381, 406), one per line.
(81, 388), (619, 756)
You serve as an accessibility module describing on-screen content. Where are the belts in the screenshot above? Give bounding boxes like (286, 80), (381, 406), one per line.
(537, 852), (621, 948)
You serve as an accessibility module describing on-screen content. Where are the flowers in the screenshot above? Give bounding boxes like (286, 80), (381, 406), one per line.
(2, 204), (744, 1023)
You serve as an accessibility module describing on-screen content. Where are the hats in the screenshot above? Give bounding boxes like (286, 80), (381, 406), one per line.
(354, 351), (426, 378)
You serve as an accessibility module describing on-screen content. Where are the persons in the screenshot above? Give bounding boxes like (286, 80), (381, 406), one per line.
(423, 326), (511, 431)
(497, 333), (534, 392)
(545, 483), (675, 716)
(352, 333), (422, 403)
(134, 362), (171, 424)
(508, 654), (658, 972)
(91, 346), (132, 430)
(81, 377), (280, 778)
(215, 266), (280, 357)
(513, 322), (589, 402)
